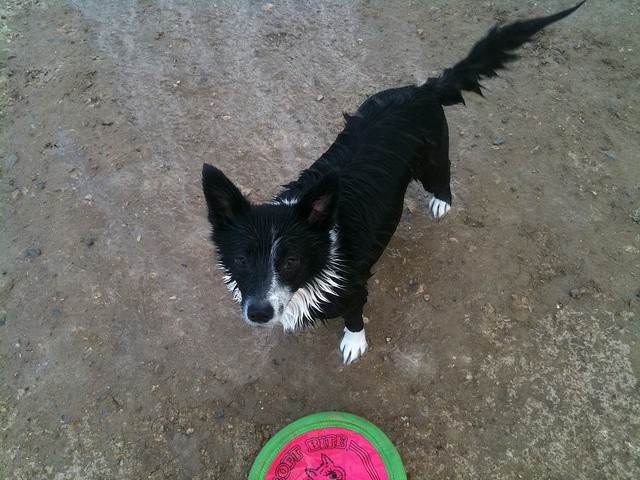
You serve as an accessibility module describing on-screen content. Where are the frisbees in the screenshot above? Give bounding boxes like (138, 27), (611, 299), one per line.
(247, 412), (409, 480)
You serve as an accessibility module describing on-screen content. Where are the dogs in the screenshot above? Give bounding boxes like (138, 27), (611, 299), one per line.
(201, 0), (588, 364)
